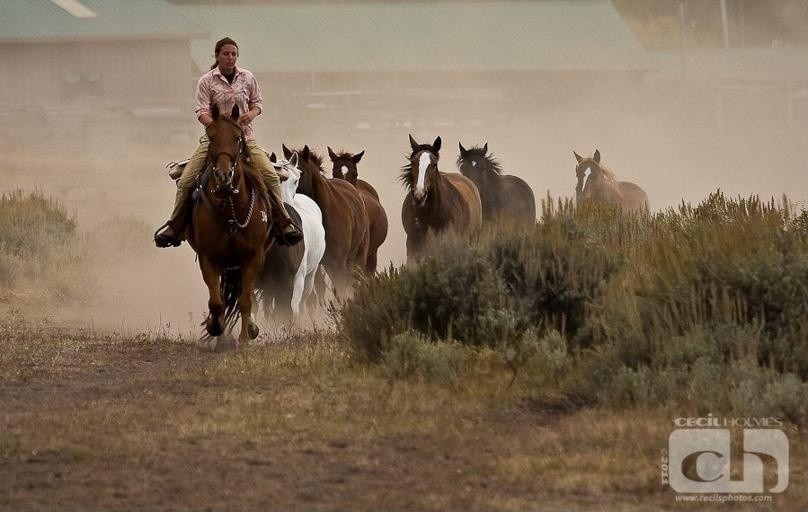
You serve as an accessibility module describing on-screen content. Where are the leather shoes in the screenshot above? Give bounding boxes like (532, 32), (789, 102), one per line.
(276, 230), (304, 246)
(155, 225), (185, 248)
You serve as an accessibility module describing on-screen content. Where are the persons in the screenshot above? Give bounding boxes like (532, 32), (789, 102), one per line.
(154, 37), (304, 247)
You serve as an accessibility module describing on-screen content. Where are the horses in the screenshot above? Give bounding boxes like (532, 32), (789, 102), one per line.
(397, 134), (484, 263)
(177, 97), (390, 341)
(453, 141), (535, 240)
(570, 147), (652, 223)
